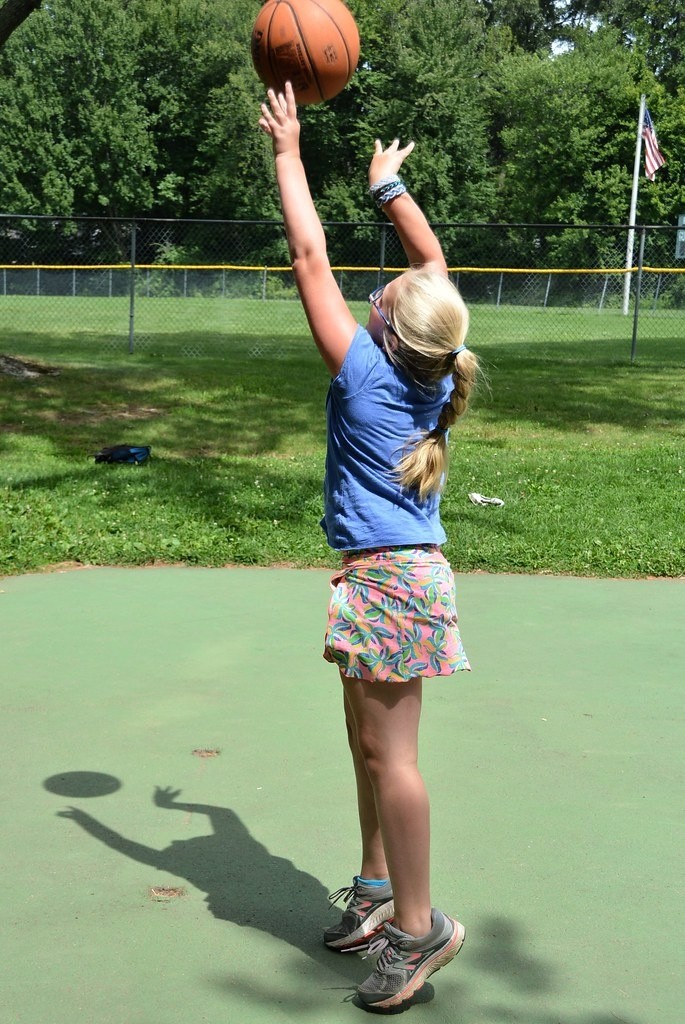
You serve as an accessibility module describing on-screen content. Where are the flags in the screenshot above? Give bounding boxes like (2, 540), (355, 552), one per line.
(642, 110), (666, 181)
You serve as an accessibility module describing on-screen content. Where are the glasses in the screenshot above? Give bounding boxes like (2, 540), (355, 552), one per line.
(368, 286), (389, 329)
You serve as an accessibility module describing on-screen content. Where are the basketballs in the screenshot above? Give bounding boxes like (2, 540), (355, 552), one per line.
(250, 1), (360, 107)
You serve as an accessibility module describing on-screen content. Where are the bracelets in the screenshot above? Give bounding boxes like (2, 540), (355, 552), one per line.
(369, 173), (406, 208)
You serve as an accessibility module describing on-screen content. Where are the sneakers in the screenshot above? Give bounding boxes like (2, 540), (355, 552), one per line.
(355, 908), (465, 1011)
(323, 875), (398, 949)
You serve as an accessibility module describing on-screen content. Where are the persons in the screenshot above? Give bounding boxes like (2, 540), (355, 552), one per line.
(256, 80), (483, 1009)
